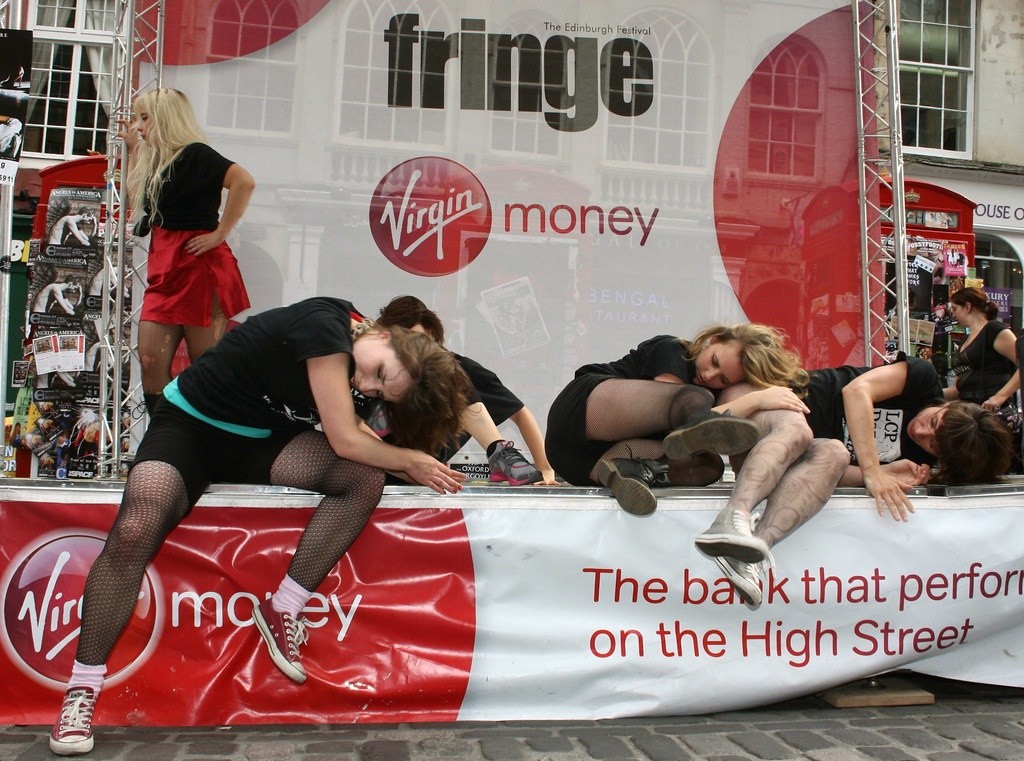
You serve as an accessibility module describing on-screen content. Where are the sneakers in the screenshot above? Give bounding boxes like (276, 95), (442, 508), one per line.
(49, 680), (104, 757)
(488, 440), (544, 487)
(713, 557), (763, 607)
(250, 596), (307, 685)
(693, 507), (770, 564)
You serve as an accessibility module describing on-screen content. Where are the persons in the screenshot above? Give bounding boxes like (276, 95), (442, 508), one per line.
(44, 297), (466, 756)
(33, 205), (129, 316)
(544, 323), (813, 518)
(693, 350), (1012, 611)
(939, 285), (1024, 474)
(114, 88), (256, 425)
(364, 295), (564, 486)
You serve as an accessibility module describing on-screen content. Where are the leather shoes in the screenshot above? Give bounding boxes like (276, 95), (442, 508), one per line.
(662, 408), (760, 461)
(596, 456), (674, 516)
